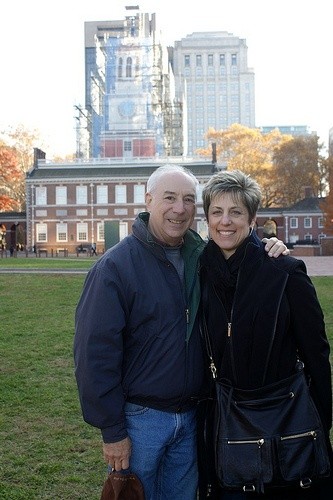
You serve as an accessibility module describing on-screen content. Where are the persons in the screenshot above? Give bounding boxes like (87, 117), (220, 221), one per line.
(74, 164), (291, 500)
(199, 169), (332, 500)
(91, 243), (97, 256)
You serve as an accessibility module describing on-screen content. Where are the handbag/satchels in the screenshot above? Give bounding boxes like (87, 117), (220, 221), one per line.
(199, 304), (333, 489)
(101, 470), (146, 500)
